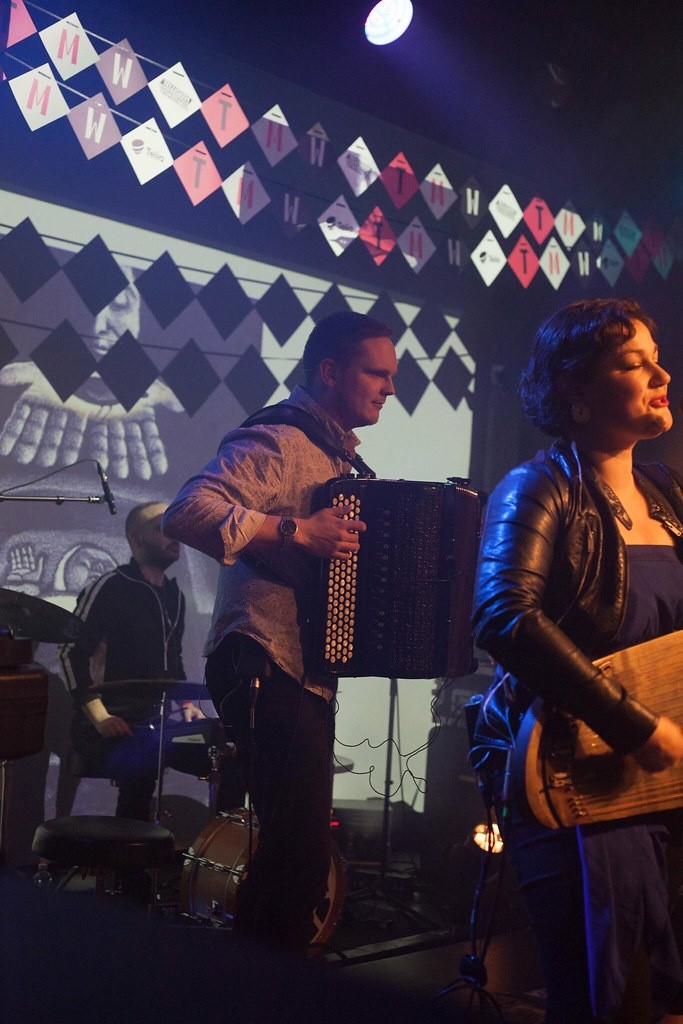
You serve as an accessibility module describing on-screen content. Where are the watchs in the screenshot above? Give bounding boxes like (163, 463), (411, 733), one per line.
(278, 515), (298, 557)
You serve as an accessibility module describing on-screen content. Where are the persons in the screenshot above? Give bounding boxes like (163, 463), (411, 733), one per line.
(159, 312), (398, 953)
(464, 296), (682, 1024)
(54, 501), (246, 827)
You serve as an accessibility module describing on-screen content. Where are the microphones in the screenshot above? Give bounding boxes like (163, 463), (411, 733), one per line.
(97, 463), (117, 515)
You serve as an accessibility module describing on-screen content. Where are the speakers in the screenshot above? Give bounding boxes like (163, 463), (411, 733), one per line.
(423, 726), (507, 898)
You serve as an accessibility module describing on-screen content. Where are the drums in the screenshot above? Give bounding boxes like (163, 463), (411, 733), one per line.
(178, 806), (347, 958)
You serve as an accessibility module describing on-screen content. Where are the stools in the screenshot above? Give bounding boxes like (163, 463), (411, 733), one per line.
(57, 704), (169, 819)
(31, 815), (175, 919)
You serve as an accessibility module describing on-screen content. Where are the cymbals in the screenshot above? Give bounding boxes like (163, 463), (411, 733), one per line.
(0, 588), (84, 644)
(87, 679), (212, 701)
